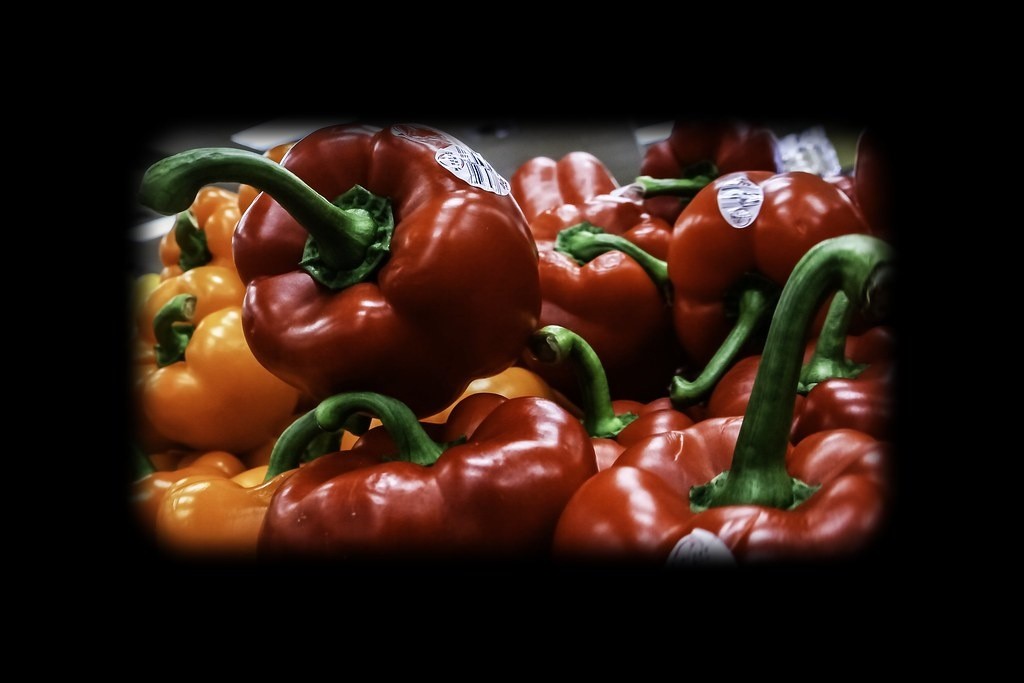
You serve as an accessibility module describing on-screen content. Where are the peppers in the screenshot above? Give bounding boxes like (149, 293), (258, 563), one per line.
(130, 123), (891, 559)
